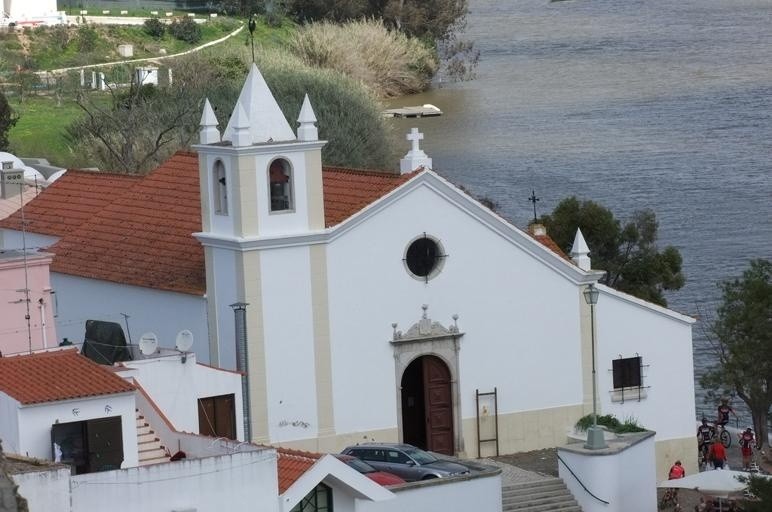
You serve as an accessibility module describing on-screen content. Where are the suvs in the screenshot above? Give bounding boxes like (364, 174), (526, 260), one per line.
(335, 441), (472, 483)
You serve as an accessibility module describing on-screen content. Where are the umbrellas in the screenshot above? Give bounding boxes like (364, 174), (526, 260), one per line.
(657, 469), (772, 511)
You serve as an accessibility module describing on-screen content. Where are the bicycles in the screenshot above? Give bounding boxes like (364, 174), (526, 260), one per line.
(698, 443), (709, 465)
(662, 489), (680, 508)
(713, 420), (731, 449)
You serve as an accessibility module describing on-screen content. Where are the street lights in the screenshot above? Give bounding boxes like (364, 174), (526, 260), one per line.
(584, 282), (608, 449)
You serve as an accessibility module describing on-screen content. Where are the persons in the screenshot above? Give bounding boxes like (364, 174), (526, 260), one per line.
(662, 396), (758, 511)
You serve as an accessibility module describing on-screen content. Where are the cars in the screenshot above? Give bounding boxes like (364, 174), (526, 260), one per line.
(324, 455), (409, 489)
